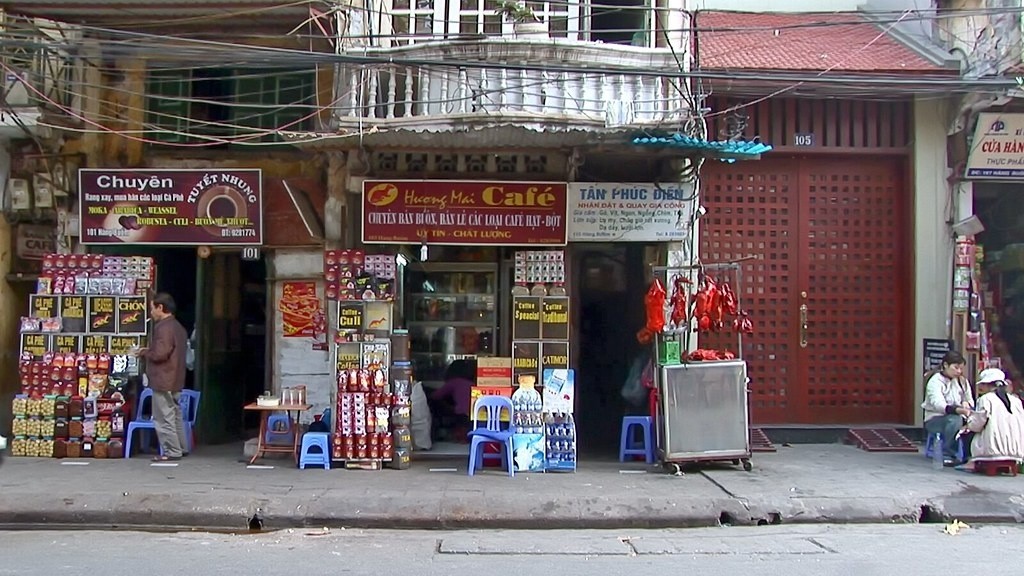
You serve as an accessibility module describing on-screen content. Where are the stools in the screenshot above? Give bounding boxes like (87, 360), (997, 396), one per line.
(264, 413), (294, 446)
(618, 415), (657, 463)
(298, 431), (332, 469)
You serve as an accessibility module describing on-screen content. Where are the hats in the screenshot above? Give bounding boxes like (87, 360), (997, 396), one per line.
(975, 368), (1008, 386)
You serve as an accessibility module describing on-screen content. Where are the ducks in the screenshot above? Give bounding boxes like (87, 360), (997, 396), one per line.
(645, 274), (753, 360)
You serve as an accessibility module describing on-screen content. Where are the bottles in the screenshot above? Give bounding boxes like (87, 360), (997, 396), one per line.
(514, 394), (575, 462)
(281, 385), (306, 405)
(12, 393), (127, 458)
(389, 330), (413, 468)
(511, 281), (568, 297)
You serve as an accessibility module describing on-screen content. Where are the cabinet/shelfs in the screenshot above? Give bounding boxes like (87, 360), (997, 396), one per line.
(403, 261), (499, 391)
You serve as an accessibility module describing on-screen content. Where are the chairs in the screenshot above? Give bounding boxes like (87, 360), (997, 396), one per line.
(469, 394), (513, 477)
(125, 388), (201, 458)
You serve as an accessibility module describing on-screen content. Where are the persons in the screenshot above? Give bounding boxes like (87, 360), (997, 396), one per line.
(133, 293), (189, 463)
(920, 350), (975, 467)
(967, 367), (1024, 462)
(427, 359), (477, 442)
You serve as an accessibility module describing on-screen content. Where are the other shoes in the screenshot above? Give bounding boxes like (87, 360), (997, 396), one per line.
(151, 455), (180, 461)
(182, 452), (189, 457)
(955, 463), (975, 473)
(944, 459), (954, 467)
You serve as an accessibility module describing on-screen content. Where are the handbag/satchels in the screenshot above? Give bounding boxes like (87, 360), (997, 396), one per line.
(641, 358), (653, 388)
(622, 357), (648, 411)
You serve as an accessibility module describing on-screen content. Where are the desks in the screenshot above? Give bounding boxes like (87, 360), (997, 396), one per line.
(243, 402), (314, 465)
